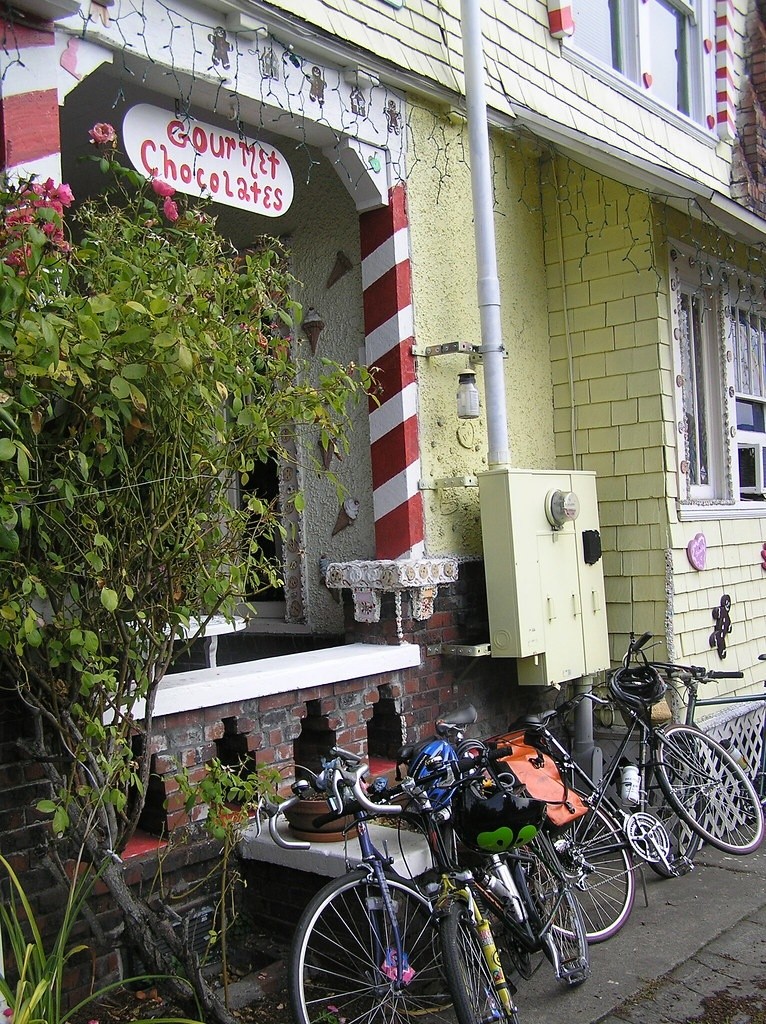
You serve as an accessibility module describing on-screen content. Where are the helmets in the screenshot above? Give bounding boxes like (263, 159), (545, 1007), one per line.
(451, 788), (547, 854)
(407, 739), (465, 813)
(608, 664), (668, 713)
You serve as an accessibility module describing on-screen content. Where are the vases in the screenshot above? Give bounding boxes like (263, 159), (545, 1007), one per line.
(282, 791), (357, 843)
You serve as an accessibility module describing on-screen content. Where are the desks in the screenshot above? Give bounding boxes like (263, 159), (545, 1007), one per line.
(126, 614), (247, 668)
(240, 812), (435, 992)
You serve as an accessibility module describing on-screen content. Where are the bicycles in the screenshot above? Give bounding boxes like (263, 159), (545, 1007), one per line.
(251, 632), (766, 1024)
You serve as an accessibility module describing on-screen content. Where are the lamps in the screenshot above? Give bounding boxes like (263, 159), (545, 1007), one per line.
(456, 369), (479, 419)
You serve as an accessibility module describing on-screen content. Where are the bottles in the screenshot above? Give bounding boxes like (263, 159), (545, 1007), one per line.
(490, 853), (528, 922)
(476, 872), (513, 905)
(719, 738), (752, 773)
(620, 762), (640, 806)
